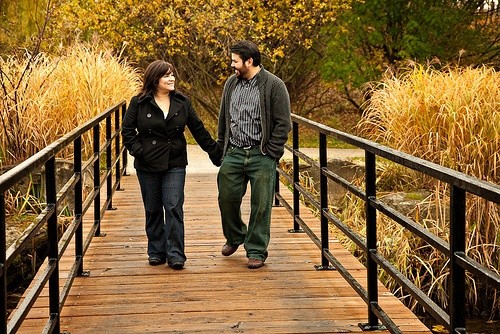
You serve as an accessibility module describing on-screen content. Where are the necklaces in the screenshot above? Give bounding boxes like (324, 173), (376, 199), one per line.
(158, 93), (169, 97)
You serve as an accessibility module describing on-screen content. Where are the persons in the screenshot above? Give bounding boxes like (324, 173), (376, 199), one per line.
(121, 60), (222, 268)
(217, 41), (292, 268)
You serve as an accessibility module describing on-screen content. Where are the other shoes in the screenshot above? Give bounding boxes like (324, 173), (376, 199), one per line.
(172, 261), (184, 266)
(149, 257), (161, 265)
(248, 258), (264, 268)
(222, 244), (239, 256)
